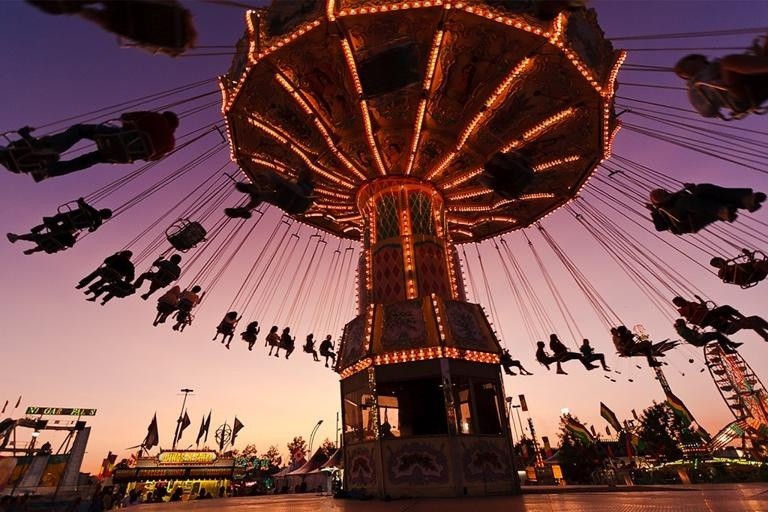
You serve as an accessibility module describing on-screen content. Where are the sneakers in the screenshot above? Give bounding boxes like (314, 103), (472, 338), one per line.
(724, 343), (743, 354)
(721, 193), (765, 221)
(224, 181), (257, 219)
(7, 232), (18, 242)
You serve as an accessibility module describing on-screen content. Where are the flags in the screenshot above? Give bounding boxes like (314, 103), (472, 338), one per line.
(562, 393), (709, 463)
(144, 410), (244, 451)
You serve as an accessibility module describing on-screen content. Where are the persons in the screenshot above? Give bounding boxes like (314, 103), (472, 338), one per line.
(90, 483), (233, 512)
(380, 422), (397, 438)
